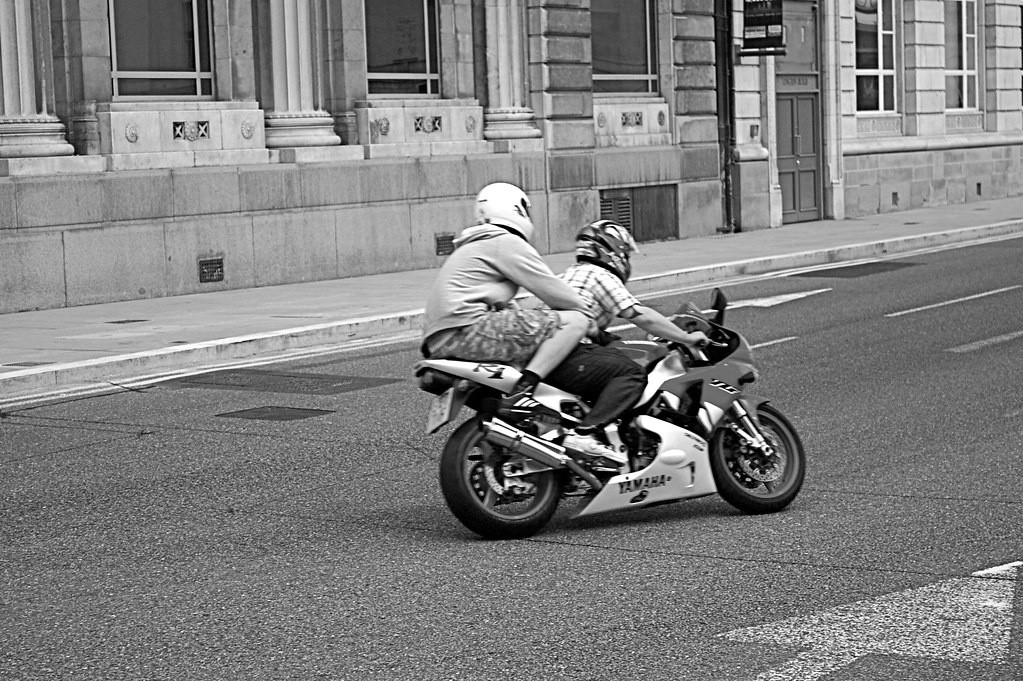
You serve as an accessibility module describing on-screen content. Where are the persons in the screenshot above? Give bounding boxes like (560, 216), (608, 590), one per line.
(420, 181), (600, 423)
(537, 218), (711, 468)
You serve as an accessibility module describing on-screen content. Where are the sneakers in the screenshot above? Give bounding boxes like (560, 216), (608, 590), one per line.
(560, 427), (614, 457)
(497, 385), (562, 424)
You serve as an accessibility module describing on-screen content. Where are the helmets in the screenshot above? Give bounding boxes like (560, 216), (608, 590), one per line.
(473, 181), (535, 241)
(575, 219), (639, 285)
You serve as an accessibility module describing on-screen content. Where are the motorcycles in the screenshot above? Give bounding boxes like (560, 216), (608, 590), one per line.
(411, 285), (808, 541)
